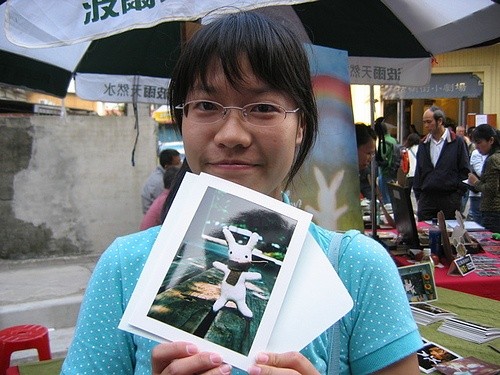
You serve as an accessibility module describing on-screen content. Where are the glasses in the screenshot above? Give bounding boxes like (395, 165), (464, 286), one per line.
(175, 99), (300, 127)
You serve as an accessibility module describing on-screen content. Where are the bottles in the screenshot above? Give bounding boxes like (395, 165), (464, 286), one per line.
(429, 218), (442, 260)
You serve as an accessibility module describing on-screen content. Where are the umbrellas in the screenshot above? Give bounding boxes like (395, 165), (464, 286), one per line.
(0, 0), (500, 240)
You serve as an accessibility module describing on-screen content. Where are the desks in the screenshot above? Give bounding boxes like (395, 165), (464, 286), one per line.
(366, 219), (500, 375)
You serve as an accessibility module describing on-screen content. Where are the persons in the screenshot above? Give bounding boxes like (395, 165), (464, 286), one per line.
(57, 8), (426, 375)
(355, 107), (500, 234)
(140, 149), (181, 231)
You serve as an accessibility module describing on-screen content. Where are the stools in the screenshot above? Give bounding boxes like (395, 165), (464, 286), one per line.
(0, 325), (51, 375)
